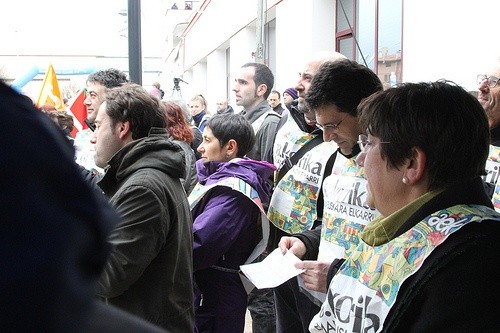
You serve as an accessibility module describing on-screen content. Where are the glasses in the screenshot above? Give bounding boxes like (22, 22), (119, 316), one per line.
(315, 111), (352, 133)
(357, 135), (393, 152)
(476, 74), (500, 88)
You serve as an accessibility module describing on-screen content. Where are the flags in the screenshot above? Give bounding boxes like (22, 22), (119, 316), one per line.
(65, 88), (89, 138)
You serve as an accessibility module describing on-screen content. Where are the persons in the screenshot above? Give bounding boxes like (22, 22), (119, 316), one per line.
(0, 51), (500, 333)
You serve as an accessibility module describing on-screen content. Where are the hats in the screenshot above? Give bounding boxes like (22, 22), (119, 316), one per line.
(284, 88), (298, 100)
